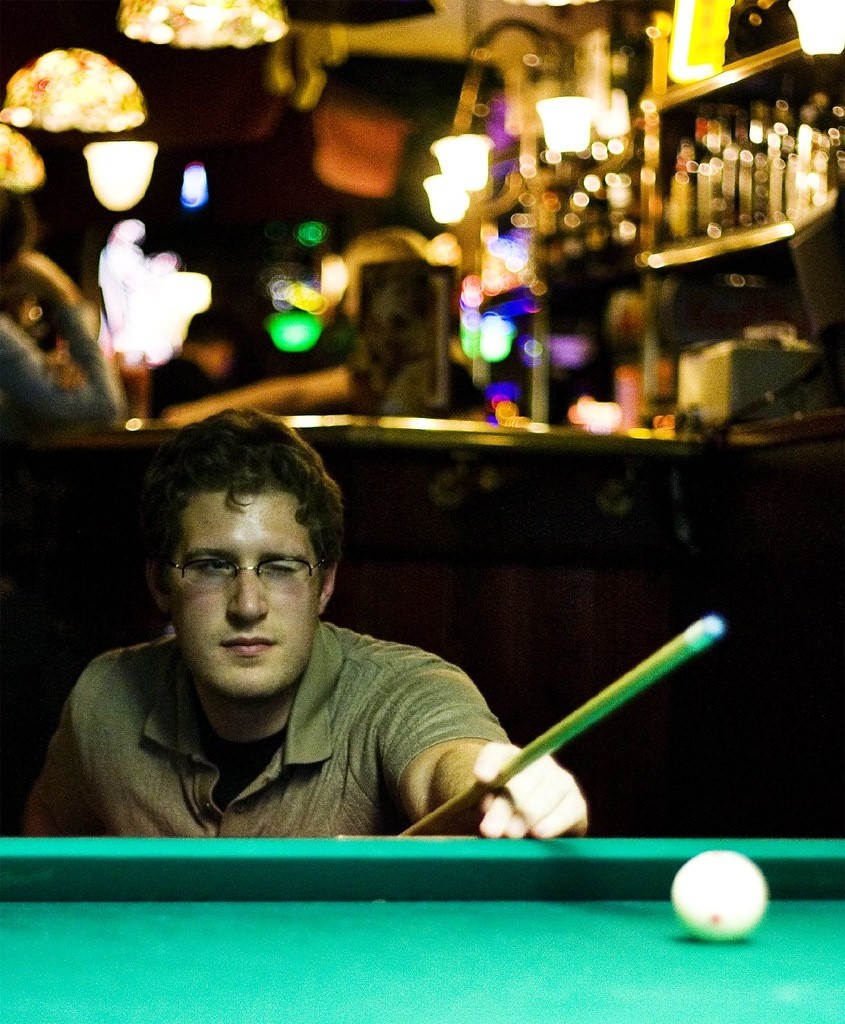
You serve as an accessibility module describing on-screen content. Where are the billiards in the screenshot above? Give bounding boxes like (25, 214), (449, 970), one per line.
(671, 851), (771, 943)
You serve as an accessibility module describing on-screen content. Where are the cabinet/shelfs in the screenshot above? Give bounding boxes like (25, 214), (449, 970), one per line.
(463, 39), (845, 424)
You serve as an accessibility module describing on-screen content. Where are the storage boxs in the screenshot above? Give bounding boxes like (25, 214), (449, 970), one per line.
(678, 337), (813, 426)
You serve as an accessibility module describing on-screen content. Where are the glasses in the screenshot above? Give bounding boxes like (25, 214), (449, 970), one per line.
(166, 557), (326, 592)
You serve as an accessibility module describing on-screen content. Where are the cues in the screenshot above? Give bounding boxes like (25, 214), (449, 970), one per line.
(393, 614), (728, 837)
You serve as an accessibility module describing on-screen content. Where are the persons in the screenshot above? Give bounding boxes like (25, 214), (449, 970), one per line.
(0, 188), (234, 421)
(25, 405), (588, 839)
(340, 223), (489, 426)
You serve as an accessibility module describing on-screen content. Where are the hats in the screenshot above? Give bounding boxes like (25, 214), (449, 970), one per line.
(325, 51), (436, 127)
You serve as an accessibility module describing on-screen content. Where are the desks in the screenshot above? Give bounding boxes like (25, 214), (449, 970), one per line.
(0, 833), (845, 1024)
